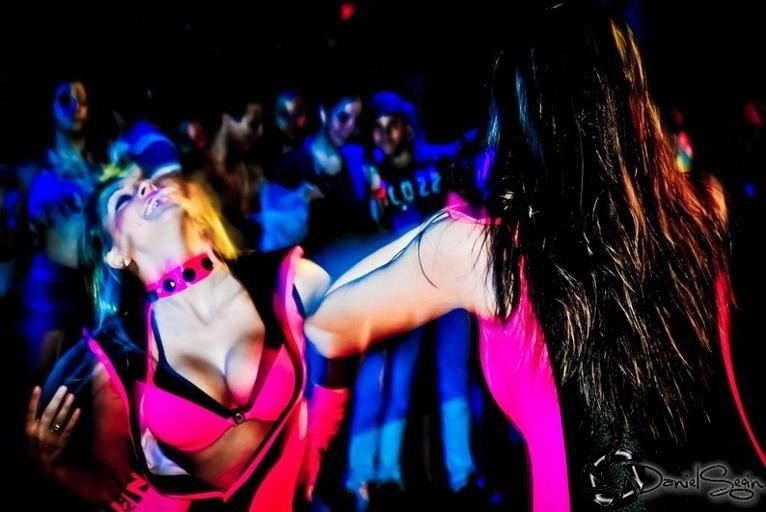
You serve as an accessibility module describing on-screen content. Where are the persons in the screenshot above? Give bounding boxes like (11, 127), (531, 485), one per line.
(0, 0), (765, 511)
